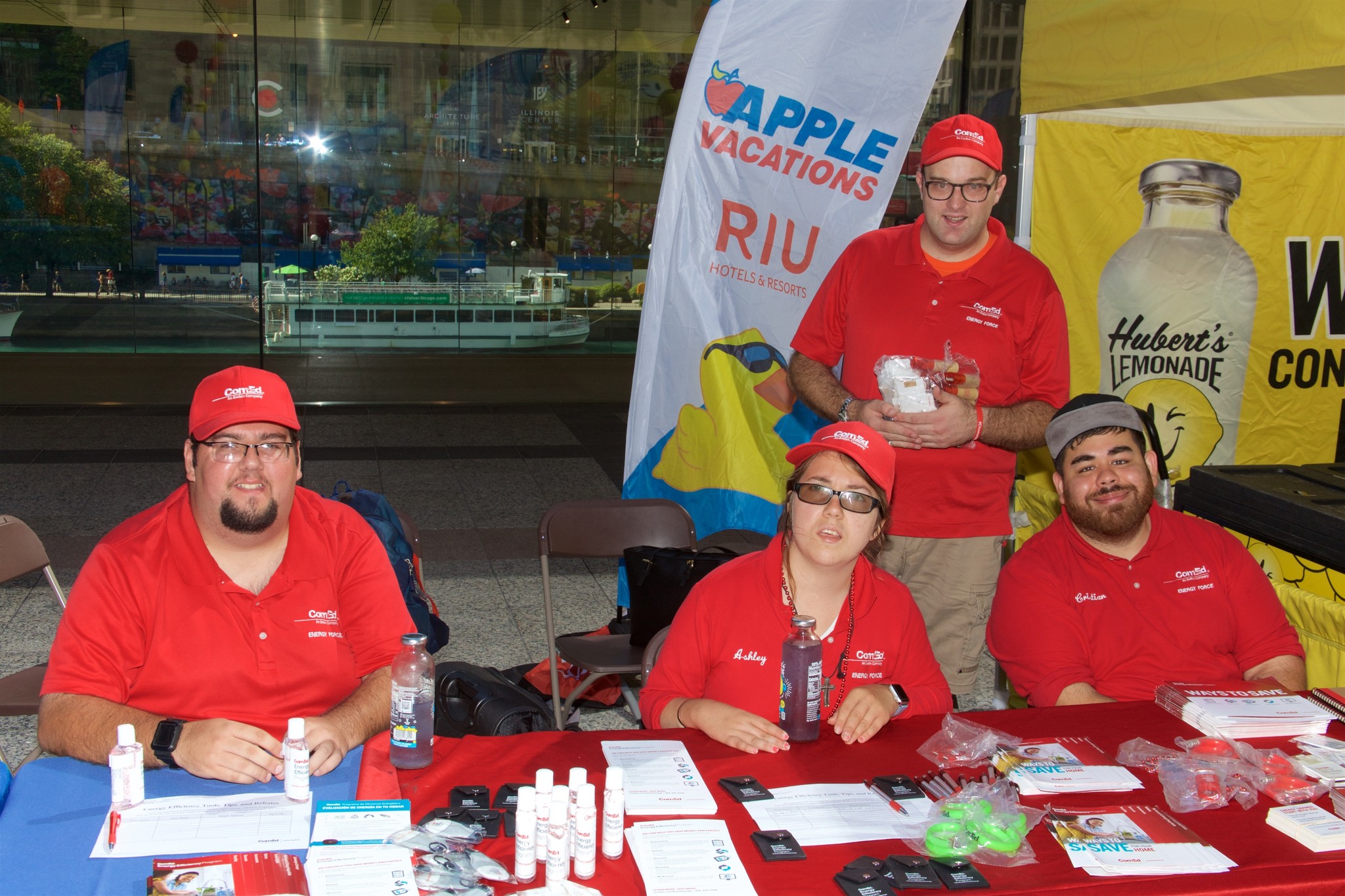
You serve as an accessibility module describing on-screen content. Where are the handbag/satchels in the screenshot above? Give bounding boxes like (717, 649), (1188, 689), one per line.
(623, 546), (741, 647)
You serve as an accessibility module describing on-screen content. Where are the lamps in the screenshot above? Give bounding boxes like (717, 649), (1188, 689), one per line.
(591, 0), (599, 9)
(562, 10), (570, 24)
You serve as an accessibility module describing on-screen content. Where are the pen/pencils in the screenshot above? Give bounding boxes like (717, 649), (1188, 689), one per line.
(956, 773), (967, 791)
(109, 810), (122, 852)
(863, 779), (910, 817)
(979, 772), (989, 785)
(987, 763), (997, 787)
(996, 771), (1005, 783)
(969, 777), (977, 786)
(1009, 781), (1020, 805)
(938, 767), (962, 793)
(914, 776), (945, 800)
(928, 770), (956, 796)
(922, 775), (951, 798)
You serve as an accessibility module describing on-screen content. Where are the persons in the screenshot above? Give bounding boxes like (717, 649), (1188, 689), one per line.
(86, 139), (212, 184)
(37, 365), (436, 785)
(229, 272), (249, 294)
(161, 272), (208, 295)
(20, 273), (32, 292)
(623, 276), (631, 289)
(553, 154), (586, 165)
(639, 420), (954, 754)
(96, 269), (116, 295)
(789, 112), (1072, 711)
(53, 271), (63, 293)
(265, 133), (286, 147)
(986, 393), (1308, 707)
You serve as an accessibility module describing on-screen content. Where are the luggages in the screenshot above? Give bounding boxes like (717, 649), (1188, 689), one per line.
(413, 662), (556, 734)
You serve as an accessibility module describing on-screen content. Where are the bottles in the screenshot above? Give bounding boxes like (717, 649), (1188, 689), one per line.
(778, 615), (822, 743)
(1096, 159), (1258, 514)
(108, 723), (145, 811)
(602, 766), (625, 860)
(389, 633), (436, 770)
(513, 763), (596, 888)
(283, 717), (310, 804)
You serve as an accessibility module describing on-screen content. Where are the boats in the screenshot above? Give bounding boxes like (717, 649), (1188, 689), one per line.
(262, 270), (591, 351)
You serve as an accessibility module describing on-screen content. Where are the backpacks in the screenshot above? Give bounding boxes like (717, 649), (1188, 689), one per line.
(325, 479), (449, 656)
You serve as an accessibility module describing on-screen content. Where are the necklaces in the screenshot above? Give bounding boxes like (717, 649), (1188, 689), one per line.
(781, 544), (855, 719)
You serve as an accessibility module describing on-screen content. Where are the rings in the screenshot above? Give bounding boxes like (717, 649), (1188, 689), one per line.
(888, 440), (892, 446)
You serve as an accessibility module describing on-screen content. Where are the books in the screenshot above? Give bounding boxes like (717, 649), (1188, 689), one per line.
(1307, 686), (1345, 724)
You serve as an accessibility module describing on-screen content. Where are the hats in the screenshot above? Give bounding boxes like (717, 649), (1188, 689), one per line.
(786, 421), (896, 504)
(188, 364), (302, 440)
(1043, 391), (1145, 468)
(920, 114), (1003, 174)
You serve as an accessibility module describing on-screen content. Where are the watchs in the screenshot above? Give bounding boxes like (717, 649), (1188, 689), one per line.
(150, 717), (187, 770)
(888, 683), (910, 718)
(837, 396), (857, 422)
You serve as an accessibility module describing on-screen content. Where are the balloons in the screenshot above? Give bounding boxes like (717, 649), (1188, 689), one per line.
(637, 63), (690, 163)
(178, 32), (231, 185)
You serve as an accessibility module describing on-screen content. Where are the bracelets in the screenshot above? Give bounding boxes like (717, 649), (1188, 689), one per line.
(677, 698), (693, 728)
(973, 405), (984, 441)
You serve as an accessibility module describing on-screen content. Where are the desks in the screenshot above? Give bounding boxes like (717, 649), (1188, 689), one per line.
(0, 685), (1344, 896)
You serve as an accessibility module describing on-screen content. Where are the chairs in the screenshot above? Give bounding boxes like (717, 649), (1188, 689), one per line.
(538, 499), (772, 732)
(0, 514), (69, 774)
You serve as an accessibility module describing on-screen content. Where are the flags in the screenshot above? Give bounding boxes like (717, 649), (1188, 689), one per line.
(82, 40), (129, 174)
(621, 0), (973, 533)
(421, 48), (544, 264)
(584, 291), (587, 304)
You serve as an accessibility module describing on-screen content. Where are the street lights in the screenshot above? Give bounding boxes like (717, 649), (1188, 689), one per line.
(310, 234), (319, 280)
(510, 240), (518, 283)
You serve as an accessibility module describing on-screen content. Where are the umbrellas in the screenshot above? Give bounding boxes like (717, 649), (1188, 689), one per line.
(465, 267), (486, 282)
(272, 264), (309, 274)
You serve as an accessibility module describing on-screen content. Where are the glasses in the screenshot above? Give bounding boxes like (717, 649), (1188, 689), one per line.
(190, 436), (296, 464)
(920, 170), (997, 203)
(792, 482), (880, 515)
